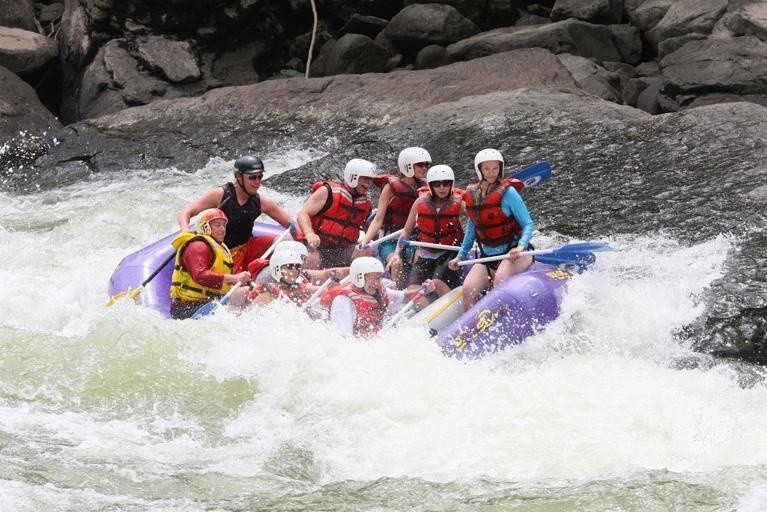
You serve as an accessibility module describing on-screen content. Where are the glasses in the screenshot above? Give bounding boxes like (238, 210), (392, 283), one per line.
(286, 264), (301, 271)
(433, 182), (450, 188)
(244, 173), (264, 181)
(416, 163), (429, 167)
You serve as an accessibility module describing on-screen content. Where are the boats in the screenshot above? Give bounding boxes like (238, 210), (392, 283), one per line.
(108, 221), (577, 360)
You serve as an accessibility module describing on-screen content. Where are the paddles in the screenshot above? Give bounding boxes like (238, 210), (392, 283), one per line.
(455, 242), (606, 267)
(107, 238), (180, 308)
(397, 235), (596, 267)
(191, 222), (296, 321)
(359, 162), (552, 248)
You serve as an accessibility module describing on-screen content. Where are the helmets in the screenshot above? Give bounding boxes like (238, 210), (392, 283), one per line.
(343, 158), (377, 188)
(349, 255), (385, 289)
(232, 155), (266, 175)
(474, 149), (505, 182)
(397, 146), (433, 179)
(269, 250), (304, 283)
(193, 208), (228, 237)
(426, 164), (455, 195)
(274, 240), (309, 259)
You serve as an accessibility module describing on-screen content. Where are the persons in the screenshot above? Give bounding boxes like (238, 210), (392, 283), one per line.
(448, 148), (536, 311)
(247, 248), (343, 320)
(385, 164), (468, 314)
(174, 154), (304, 276)
(330, 256), (436, 337)
(297, 158), (377, 269)
(356, 146), (432, 289)
(275, 240), (349, 284)
(170, 207), (251, 320)
